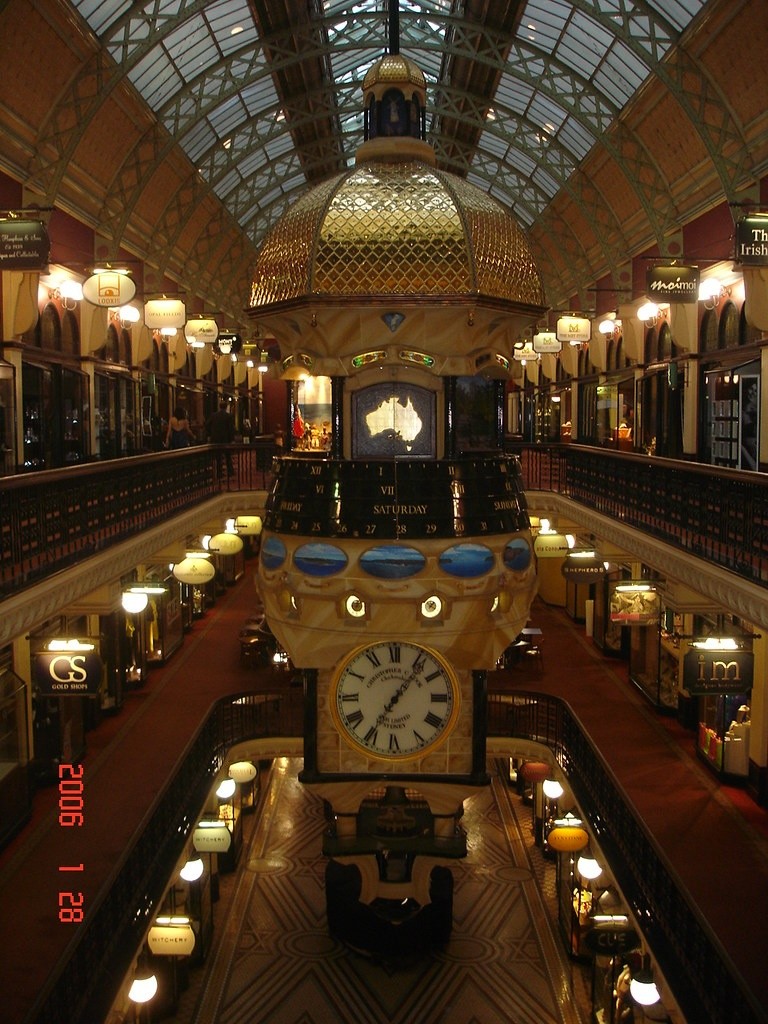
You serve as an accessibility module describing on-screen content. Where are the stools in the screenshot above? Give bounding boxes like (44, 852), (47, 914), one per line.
(237, 602), (264, 667)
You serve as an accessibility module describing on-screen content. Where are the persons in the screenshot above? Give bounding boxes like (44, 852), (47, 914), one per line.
(166, 408), (197, 450)
(206, 401), (237, 477)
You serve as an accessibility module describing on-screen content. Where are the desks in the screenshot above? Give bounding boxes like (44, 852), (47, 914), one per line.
(232, 694), (282, 722)
(488, 695), (539, 729)
(521, 628), (542, 650)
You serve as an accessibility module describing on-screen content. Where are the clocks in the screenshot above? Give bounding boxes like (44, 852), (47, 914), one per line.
(329, 640), (461, 763)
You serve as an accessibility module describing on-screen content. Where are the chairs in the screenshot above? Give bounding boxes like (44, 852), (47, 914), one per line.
(525, 639), (545, 674)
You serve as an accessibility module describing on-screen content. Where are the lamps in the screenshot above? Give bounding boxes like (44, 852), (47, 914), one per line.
(110, 306), (140, 330)
(216, 774), (236, 798)
(129, 957), (157, 1002)
(630, 954), (660, 1005)
(543, 768), (563, 798)
(49, 281), (84, 311)
(599, 320), (619, 340)
(577, 846), (602, 879)
(637, 303), (667, 328)
(153, 328), (177, 343)
(698, 278), (731, 310)
(180, 850), (204, 882)
(570, 341), (587, 350)
